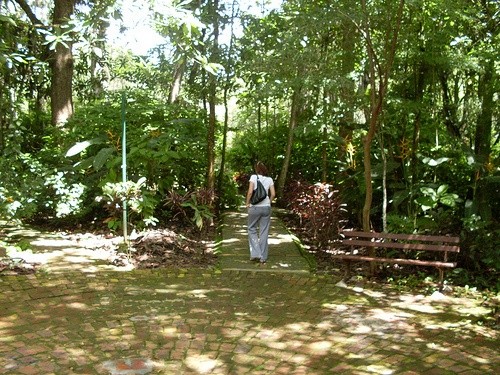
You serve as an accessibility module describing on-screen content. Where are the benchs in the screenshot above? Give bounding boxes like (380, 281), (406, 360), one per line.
(333, 231), (460, 294)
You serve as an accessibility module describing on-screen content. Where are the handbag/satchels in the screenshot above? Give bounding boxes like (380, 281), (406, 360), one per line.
(250, 174), (266, 204)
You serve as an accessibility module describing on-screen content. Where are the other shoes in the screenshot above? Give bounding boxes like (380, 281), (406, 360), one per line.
(250, 256), (266, 263)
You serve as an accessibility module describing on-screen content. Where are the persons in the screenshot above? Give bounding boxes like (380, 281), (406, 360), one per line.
(246, 162), (276, 262)
